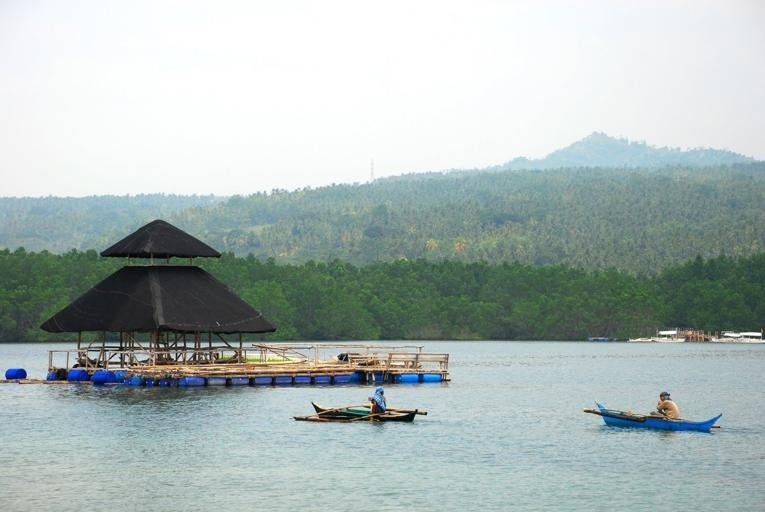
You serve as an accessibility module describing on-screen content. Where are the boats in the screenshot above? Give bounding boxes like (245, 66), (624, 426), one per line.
(589, 328), (765, 344)
(594, 400), (723, 432)
(309, 401), (419, 423)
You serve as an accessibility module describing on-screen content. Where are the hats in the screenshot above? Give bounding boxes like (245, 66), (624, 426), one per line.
(660, 392), (670, 396)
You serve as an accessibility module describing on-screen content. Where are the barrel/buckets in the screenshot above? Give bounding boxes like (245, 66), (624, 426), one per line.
(43, 367), (445, 388)
(5, 367), (27, 379)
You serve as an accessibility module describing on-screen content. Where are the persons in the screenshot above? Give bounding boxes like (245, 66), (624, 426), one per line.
(649, 391), (680, 419)
(368, 386), (386, 414)
